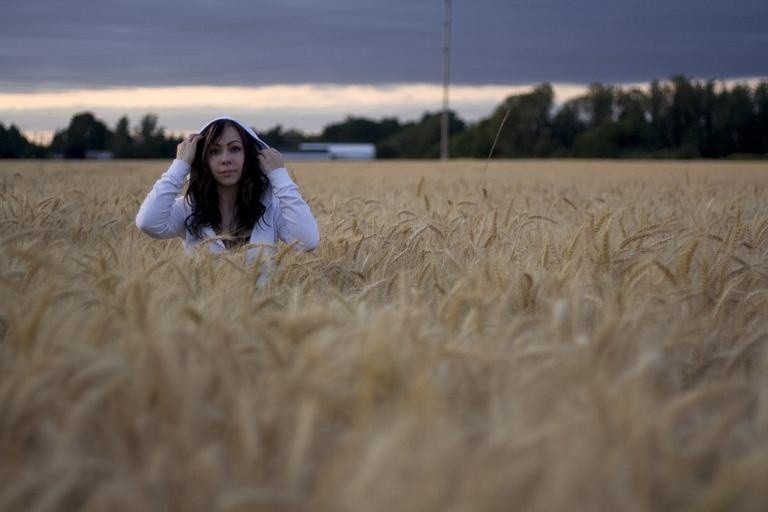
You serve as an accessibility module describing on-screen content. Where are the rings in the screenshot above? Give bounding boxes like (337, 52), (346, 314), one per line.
(186, 137), (192, 141)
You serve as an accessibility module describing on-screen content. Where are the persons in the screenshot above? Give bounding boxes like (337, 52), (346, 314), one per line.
(134, 116), (320, 291)
